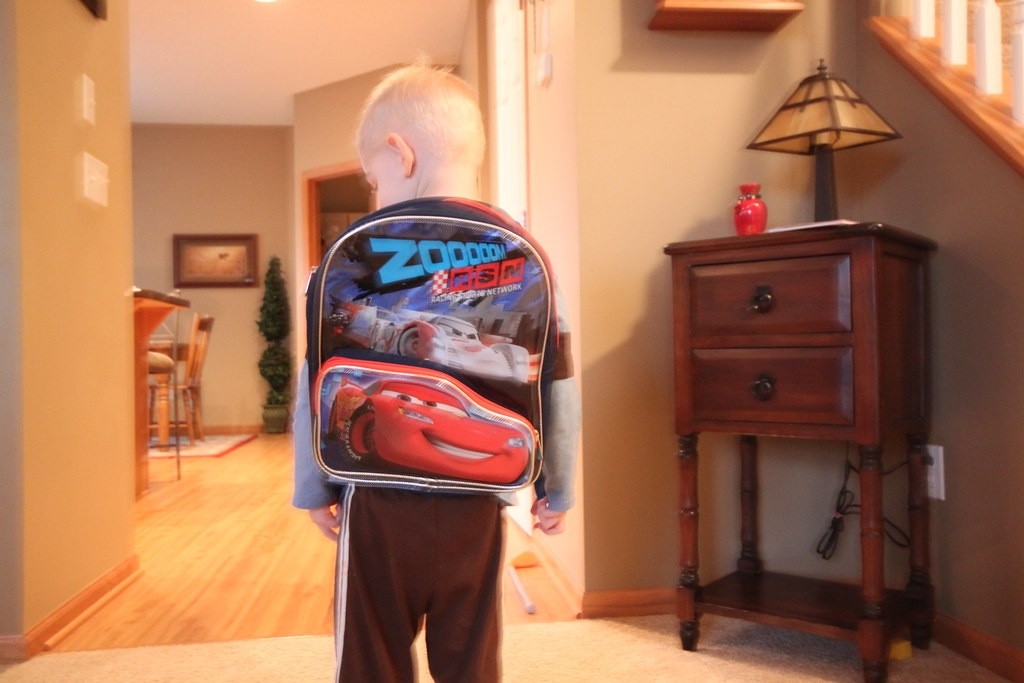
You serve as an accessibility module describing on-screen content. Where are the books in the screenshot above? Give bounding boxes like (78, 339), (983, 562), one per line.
(770, 219), (859, 232)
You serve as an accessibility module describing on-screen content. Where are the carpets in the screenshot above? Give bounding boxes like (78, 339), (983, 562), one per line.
(148, 433), (259, 459)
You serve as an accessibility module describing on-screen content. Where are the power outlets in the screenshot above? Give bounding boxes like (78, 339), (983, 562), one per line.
(926, 444), (946, 501)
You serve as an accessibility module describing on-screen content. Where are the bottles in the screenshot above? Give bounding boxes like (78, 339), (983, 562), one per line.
(734, 183), (769, 234)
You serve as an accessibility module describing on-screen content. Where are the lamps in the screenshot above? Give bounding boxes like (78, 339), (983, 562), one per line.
(745, 57), (903, 234)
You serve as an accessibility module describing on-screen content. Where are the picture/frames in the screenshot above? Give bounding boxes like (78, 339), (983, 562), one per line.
(171, 232), (260, 289)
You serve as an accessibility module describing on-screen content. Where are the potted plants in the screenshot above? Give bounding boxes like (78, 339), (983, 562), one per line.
(254, 255), (293, 434)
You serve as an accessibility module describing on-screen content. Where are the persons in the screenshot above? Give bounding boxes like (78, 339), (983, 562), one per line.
(293, 65), (579, 683)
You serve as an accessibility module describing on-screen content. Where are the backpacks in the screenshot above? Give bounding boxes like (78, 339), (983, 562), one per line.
(302, 196), (559, 493)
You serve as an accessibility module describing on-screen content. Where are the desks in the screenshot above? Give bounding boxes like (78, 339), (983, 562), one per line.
(151, 338), (201, 440)
(663, 221), (940, 683)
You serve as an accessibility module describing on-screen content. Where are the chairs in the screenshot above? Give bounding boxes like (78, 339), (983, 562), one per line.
(147, 350), (175, 452)
(148, 311), (215, 448)
